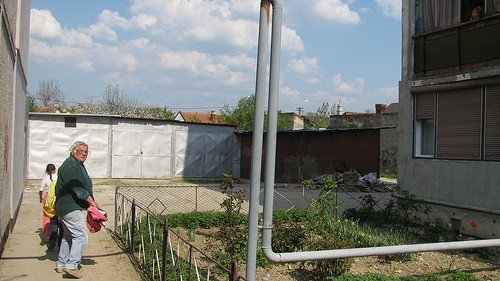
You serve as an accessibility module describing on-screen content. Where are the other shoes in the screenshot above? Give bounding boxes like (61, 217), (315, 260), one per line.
(56, 267), (65, 272)
(48, 241), (56, 249)
(40, 237), (48, 246)
(64, 268), (83, 279)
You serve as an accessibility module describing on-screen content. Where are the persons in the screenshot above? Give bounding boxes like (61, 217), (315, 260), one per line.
(38, 163), (71, 253)
(54, 140), (99, 279)
